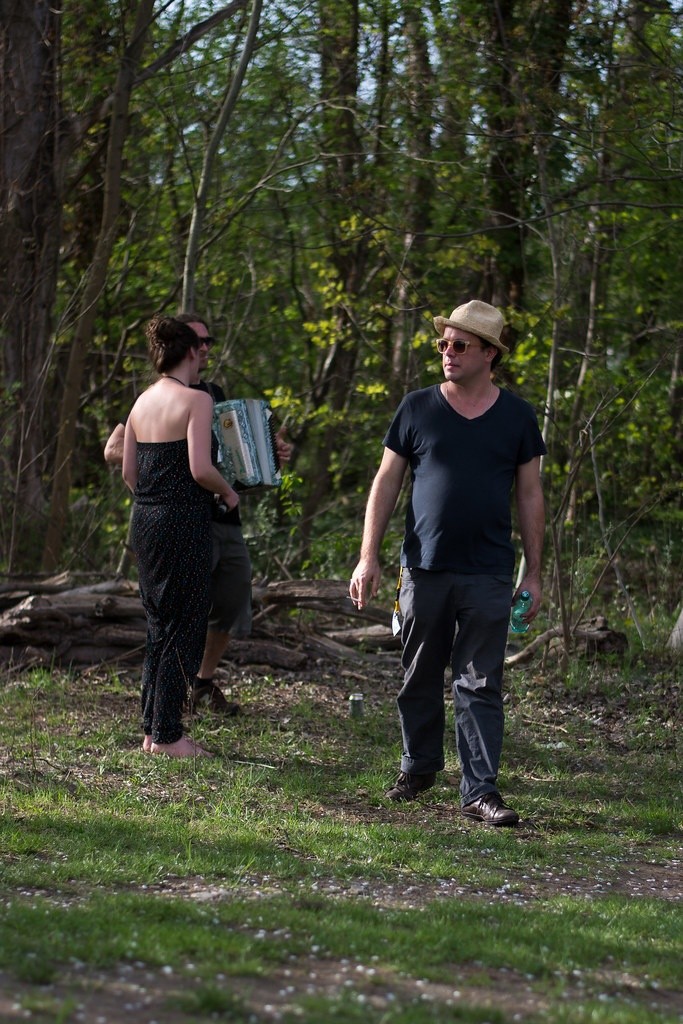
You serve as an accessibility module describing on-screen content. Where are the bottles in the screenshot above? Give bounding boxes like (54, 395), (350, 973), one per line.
(510, 591), (532, 633)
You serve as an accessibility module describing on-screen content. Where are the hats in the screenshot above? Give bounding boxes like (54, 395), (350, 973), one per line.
(433, 300), (510, 352)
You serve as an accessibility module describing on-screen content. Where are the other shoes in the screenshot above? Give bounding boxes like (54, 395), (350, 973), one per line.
(190, 682), (239, 716)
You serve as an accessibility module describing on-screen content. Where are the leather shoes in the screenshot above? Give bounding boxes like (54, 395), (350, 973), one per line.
(461, 793), (520, 824)
(385, 772), (435, 802)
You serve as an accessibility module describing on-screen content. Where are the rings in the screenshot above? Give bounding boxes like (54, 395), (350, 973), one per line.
(534, 616), (537, 620)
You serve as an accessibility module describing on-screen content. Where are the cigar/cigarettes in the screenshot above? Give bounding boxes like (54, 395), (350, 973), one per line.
(346, 596), (362, 602)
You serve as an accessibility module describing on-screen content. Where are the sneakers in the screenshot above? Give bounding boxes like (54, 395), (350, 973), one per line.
(143, 735), (203, 752)
(150, 738), (211, 757)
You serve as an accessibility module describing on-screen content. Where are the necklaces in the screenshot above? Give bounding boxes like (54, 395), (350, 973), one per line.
(164, 376), (187, 386)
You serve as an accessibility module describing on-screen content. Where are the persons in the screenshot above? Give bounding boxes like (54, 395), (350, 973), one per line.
(350, 300), (547, 823)
(103, 313), (296, 763)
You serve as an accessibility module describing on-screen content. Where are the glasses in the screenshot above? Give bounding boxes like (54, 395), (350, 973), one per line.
(436, 339), (482, 355)
(200, 337), (215, 346)
